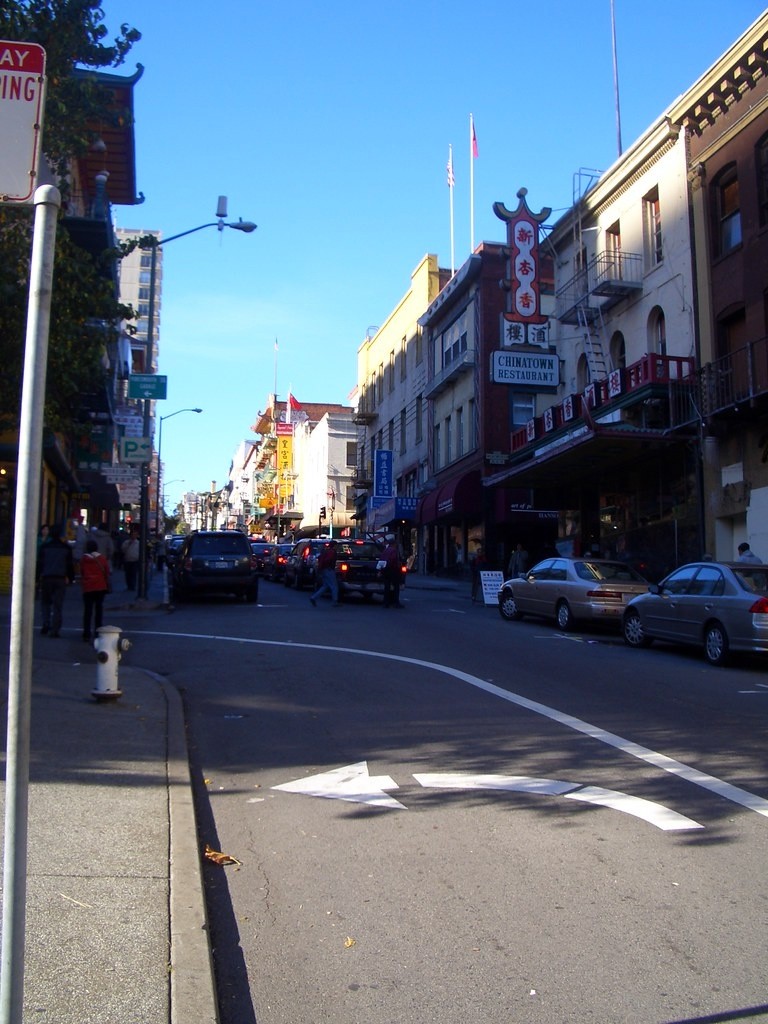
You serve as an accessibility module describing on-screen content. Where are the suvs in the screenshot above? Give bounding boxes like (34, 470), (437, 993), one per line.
(284, 538), (332, 591)
(168, 528), (257, 603)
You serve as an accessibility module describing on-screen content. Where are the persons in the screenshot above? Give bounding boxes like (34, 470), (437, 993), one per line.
(80, 540), (110, 641)
(88, 522), (140, 591)
(469, 548), (488, 601)
(156, 535), (166, 572)
(37, 523), (76, 638)
(310, 541), (342, 607)
(737, 542), (762, 565)
(377, 541), (404, 609)
(508, 544), (529, 579)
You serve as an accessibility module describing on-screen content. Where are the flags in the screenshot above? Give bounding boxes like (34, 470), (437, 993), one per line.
(290, 393), (302, 412)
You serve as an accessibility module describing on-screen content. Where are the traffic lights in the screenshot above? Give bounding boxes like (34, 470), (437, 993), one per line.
(320, 507), (326, 519)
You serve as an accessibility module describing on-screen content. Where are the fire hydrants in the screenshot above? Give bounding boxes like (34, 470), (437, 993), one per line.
(91, 624), (133, 704)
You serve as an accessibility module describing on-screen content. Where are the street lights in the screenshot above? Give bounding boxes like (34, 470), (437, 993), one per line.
(163, 480), (185, 513)
(138, 221), (257, 600)
(155, 408), (203, 564)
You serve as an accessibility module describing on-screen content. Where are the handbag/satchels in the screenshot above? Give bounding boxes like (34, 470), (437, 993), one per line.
(107, 582), (112, 594)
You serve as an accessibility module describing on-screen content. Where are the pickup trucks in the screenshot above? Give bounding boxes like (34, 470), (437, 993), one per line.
(325, 539), (406, 604)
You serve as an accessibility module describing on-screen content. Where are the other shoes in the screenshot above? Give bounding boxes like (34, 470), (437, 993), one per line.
(383, 604), (390, 608)
(310, 598), (317, 607)
(330, 603), (343, 607)
(40, 623), (49, 635)
(393, 604), (406, 609)
(50, 634), (60, 638)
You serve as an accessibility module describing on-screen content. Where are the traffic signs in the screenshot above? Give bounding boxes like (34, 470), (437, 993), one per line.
(127, 374), (168, 399)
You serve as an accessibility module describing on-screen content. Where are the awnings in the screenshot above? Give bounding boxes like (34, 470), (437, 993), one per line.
(415, 472), (481, 524)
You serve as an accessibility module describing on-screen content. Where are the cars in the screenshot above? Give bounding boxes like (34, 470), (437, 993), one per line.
(247, 536), (296, 583)
(621, 560), (768, 666)
(166, 534), (185, 566)
(498, 557), (652, 632)
